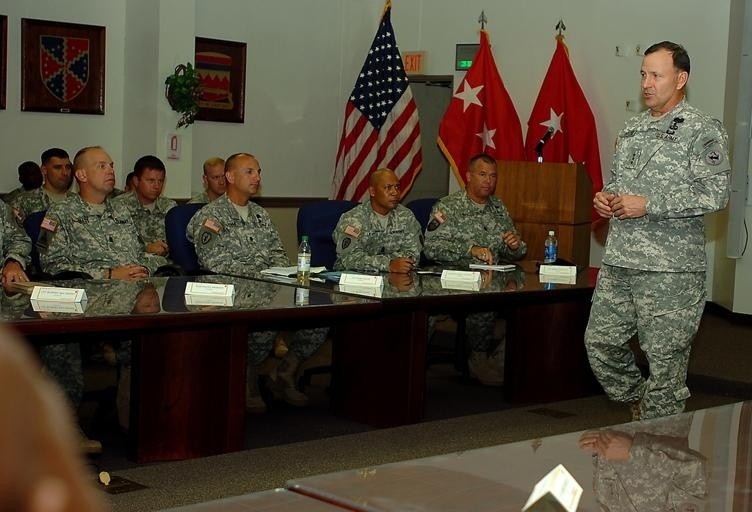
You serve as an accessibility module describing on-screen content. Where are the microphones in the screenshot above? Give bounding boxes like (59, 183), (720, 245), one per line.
(535, 127), (554, 146)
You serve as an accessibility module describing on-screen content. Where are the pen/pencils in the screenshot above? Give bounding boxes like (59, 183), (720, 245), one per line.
(398, 254), (416, 267)
(484, 262), (497, 265)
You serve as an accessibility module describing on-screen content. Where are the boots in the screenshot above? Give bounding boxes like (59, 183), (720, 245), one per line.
(246, 364), (267, 408)
(269, 351), (308, 406)
(469, 351), (505, 386)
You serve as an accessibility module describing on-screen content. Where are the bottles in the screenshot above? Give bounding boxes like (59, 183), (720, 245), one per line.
(297, 236), (311, 288)
(544, 230), (557, 265)
(294, 288), (310, 307)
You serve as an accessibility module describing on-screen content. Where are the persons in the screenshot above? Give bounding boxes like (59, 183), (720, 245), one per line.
(1, 323), (111, 510)
(1, 147), (145, 451)
(189, 157), (231, 206)
(112, 152), (191, 266)
(331, 165), (424, 275)
(422, 155), (529, 390)
(581, 40), (730, 421)
(583, 411), (713, 511)
(1, 259), (527, 322)
(185, 152), (310, 405)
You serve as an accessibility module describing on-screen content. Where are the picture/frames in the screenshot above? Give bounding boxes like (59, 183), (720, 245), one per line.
(21, 17), (106, 115)
(194, 36), (248, 124)
(0, 14), (7, 110)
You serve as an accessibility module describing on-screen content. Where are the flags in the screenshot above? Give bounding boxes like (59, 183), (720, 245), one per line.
(435, 28), (525, 200)
(526, 37), (612, 227)
(326, 2), (423, 207)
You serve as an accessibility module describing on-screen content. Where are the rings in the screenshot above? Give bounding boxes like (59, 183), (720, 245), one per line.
(481, 252), (487, 257)
